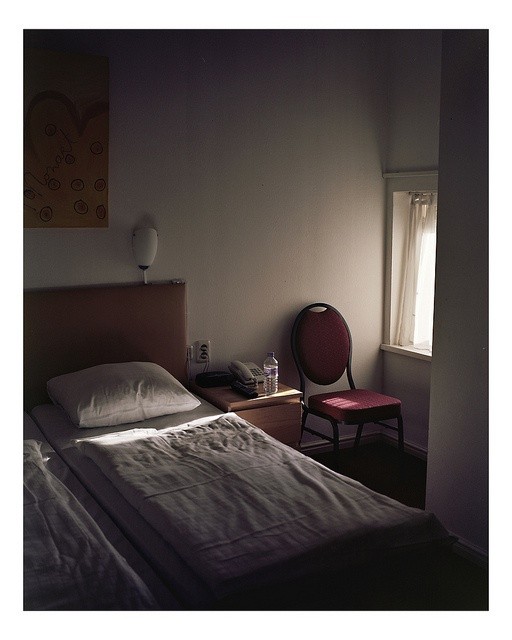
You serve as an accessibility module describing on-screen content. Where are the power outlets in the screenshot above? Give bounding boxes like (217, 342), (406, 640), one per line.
(196, 339), (210, 362)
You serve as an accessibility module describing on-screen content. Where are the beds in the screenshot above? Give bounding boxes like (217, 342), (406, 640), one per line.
(23, 281), (450, 610)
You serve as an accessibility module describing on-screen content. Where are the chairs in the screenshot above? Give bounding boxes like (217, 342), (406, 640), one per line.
(292, 303), (405, 473)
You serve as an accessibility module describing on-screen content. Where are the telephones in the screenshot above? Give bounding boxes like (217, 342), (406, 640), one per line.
(228, 360), (264, 390)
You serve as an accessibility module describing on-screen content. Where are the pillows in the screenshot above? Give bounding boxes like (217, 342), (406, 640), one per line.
(44, 361), (201, 430)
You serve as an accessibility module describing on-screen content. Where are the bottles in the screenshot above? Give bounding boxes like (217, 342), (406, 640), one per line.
(263, 352), (279, 394)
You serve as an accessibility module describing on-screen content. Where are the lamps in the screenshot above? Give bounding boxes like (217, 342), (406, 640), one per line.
(133, 228), (159, 284)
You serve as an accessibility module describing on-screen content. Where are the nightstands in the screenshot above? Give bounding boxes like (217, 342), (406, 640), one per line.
(192, 369), (304, 447)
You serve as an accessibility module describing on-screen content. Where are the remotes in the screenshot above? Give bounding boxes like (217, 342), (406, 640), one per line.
(231, 382), (258, 399)
